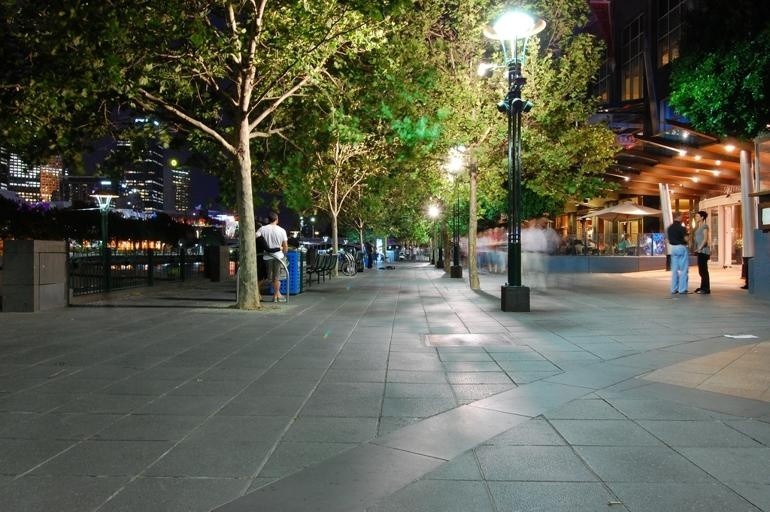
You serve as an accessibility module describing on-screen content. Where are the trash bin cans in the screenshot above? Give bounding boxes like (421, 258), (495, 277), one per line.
(269, 251), (307, 295)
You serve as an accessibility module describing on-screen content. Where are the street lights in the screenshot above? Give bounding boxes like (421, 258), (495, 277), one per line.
(470, 7), (551, 310)
(178, 239), (184, 248)
(441, 148), (470, 281)
(315, 231), (319, 238)
(427, 194), (444, 268)
(310, 217), (315, 237)
(87, 190), (119, 258)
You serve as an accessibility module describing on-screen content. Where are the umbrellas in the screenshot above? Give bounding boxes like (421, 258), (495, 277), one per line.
(576, 201), (663, 240)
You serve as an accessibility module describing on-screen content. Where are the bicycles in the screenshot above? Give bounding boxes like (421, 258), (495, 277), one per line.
(315, 250), (361, 278)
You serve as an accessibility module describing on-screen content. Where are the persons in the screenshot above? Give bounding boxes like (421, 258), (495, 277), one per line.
(639, 233), (657, 256)
(255, 222), (284, 302)
(667, 211), (690, 293)
(588, 239), (596, 246)
(457, 217), (562, 295)
(255, 212), (289, 303)
(616, 233), (633, 256)
(693, 211), (711, 295)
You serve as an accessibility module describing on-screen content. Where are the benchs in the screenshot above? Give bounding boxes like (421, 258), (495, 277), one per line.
(307, 253), (337, 286)
